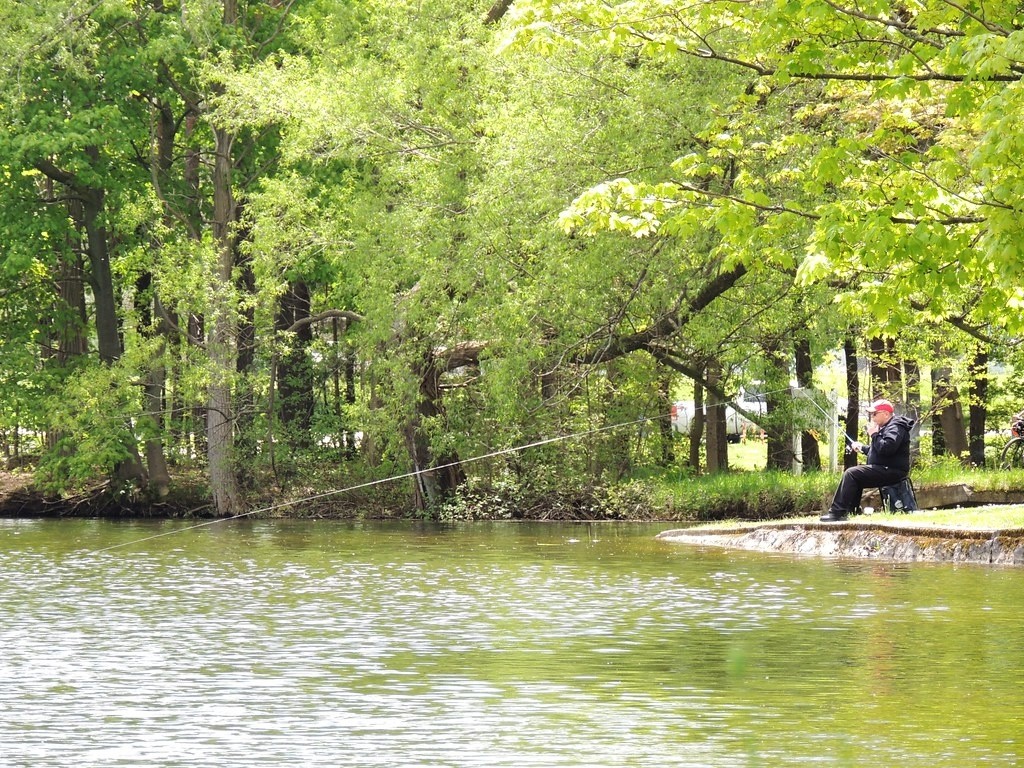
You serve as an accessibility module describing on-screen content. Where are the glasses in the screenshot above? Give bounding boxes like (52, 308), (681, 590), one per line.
(872, 412), (883, 416)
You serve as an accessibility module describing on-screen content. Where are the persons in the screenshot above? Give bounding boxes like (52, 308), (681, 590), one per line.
(819, 400), (916, 521)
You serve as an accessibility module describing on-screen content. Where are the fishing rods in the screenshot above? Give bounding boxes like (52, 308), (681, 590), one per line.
(790, 387), (858, 455)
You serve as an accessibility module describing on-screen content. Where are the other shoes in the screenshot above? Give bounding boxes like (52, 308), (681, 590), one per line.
(820, 512), (847, 522)
(847, 507), (861, 515)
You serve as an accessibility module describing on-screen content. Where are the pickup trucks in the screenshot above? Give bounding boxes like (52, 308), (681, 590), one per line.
(670, 381), (768, 439)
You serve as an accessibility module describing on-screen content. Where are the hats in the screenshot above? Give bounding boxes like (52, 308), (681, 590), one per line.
(866, 400), (894, 412)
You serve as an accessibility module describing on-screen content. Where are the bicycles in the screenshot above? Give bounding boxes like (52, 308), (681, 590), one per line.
(1001, 416), (1024, 472)
